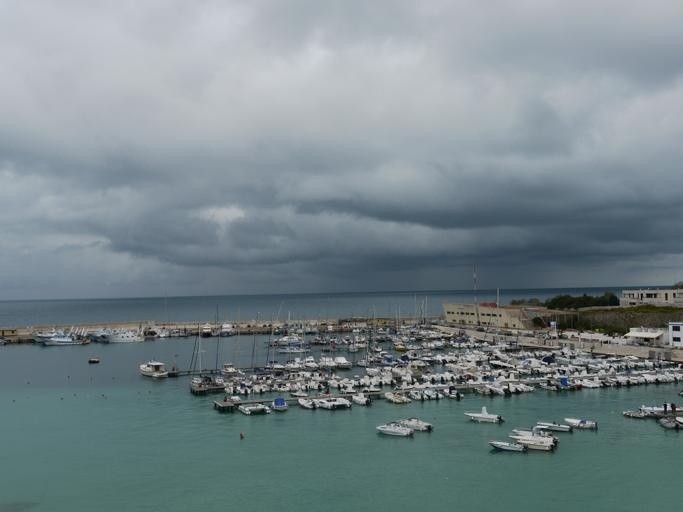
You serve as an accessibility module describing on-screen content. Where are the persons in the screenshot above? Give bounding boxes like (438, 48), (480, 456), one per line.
(663, 402), (667, 415)
(671, 403), (678, 413)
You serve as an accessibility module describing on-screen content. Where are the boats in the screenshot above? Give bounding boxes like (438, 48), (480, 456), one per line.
(507, 426), (559, 452)
(463, 406), (504, 424)
(536, 421), (572, 431)
(399, 418), (432, 432)
(564, 417), (598, 431)
(0, 294), (682, 415)
(376, 423), (413, 437)
(487, 440), (528, 453)
(619, 404), (682, 430)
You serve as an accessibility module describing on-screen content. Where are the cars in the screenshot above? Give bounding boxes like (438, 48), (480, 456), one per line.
(506, 328), (570, 340)
(477, 326), (486, 332)
